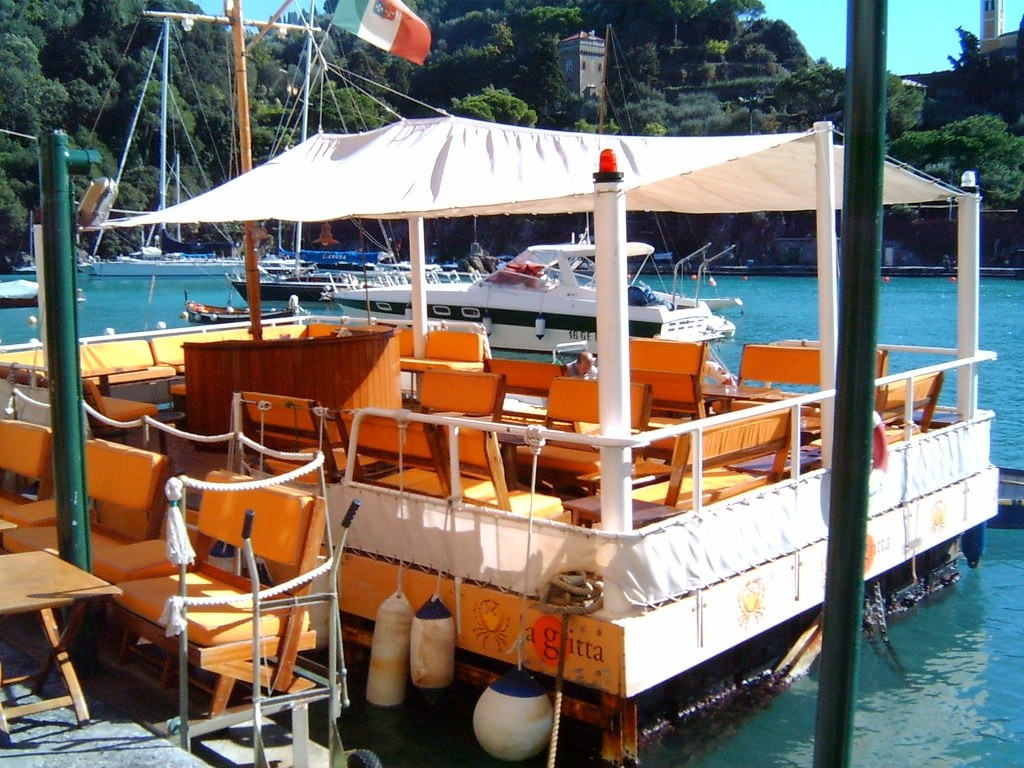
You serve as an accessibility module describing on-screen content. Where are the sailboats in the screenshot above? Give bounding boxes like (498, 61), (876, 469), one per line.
(10, 209), (39, 274)
(71, 0), (514, 304)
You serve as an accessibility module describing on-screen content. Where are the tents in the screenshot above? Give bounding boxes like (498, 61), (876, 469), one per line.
(77, 119), (980, 523)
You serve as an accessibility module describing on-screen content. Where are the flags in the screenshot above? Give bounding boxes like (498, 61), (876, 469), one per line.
(331, 0), (431, 66)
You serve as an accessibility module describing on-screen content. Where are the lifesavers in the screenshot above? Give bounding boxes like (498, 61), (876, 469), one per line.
(867, 409), (889, 497)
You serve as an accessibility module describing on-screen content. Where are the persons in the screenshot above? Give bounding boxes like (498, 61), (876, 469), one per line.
(566, 352), (597, 378)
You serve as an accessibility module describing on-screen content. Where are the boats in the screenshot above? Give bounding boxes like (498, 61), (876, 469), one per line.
(332, 211), (743, 343)
(0, 276), (88, 309)
(183, 288), (301, 327)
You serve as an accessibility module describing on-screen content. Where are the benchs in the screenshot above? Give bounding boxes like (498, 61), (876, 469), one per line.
(0, 314), (946, 768)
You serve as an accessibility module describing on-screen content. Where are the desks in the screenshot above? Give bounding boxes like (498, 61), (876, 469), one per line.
(0, 518), (18, 531)
(0, 550), (124, 748)
